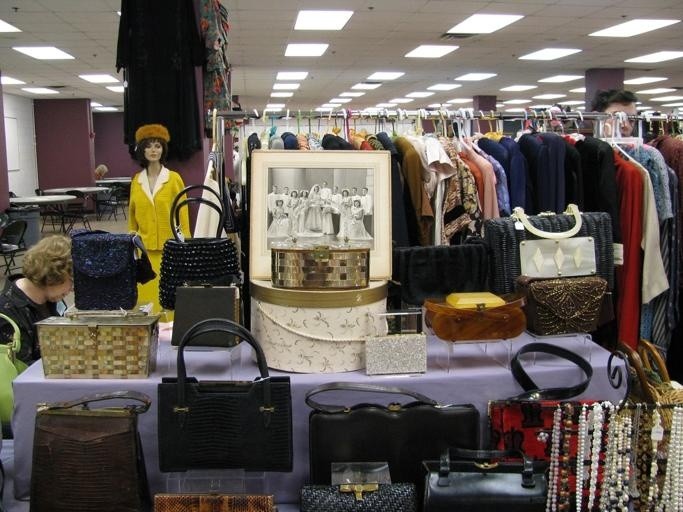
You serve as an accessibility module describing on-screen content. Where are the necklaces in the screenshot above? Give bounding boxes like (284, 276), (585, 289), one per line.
(542, 400), (682, 511)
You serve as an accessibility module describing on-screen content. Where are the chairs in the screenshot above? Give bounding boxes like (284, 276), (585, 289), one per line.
(0, 182), (126, 275)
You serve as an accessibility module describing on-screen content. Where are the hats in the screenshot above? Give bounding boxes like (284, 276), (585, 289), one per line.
(135, 124), (170, 145)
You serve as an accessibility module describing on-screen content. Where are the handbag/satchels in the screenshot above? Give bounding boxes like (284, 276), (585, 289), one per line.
(157, 318), (295, 476)
(515, 274), (613, 335)
(154, 487), (277, 512)
(305, 383), (482, 484)
(34, 308), (161, 380)
(424, 292), (527, 342)
(27, 390), (151, 511)
(159, 183), (247, 345)
(69, 228), (156, 310)
(483, 204), (617, 293)
(421, 446), (550, 511)
(487, 341), (633, 511)
(271, 246), (371, 291)
(364, 310), (427, 377)
(618, 336), (683, 511)
(0, 311), (30, 428)
(298, 480), (417, 511)
(392, 239), (492, 306)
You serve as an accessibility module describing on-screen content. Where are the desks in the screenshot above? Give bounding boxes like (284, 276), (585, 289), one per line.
(95, 177), (133, 205)
(10, 333), (627, 502)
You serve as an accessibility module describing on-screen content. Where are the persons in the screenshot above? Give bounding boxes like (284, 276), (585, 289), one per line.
(0, 233), (72, 368)
(125, 122), (193, 325)
(94, 163), (109, 180)
(269, 180), (373, 240)
(591, 86), (637, 142)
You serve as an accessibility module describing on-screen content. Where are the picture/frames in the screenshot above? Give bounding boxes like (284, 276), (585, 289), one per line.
(245, 149), (394, 282)
